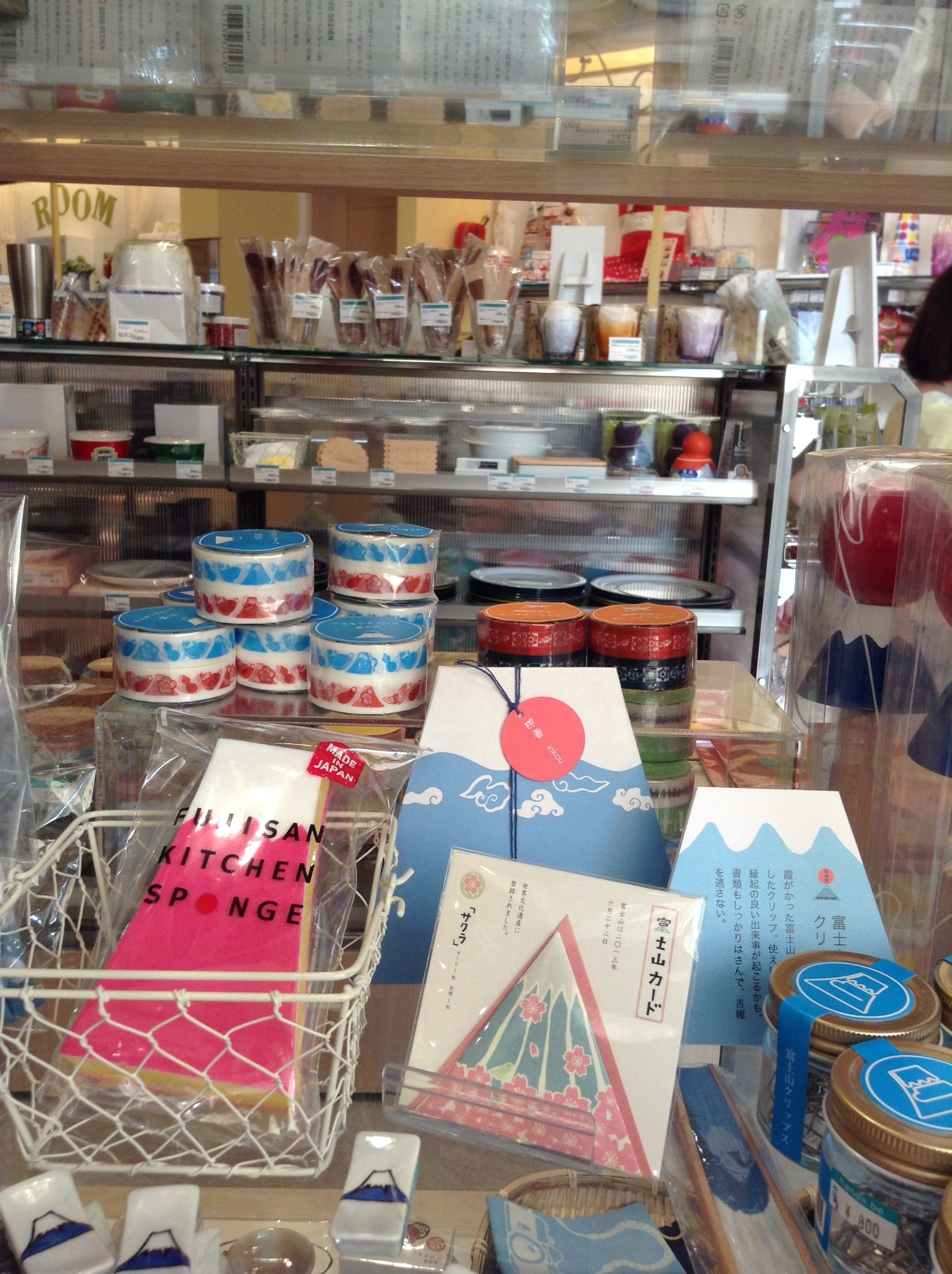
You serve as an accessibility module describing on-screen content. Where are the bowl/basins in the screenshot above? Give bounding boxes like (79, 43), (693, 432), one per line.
(225, 1227), (317, 1274)
(461, 424), (557, 472)
(0, 427), (208, 463)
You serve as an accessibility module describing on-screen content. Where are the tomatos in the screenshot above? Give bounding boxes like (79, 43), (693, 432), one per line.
(815, 479), (952, 627)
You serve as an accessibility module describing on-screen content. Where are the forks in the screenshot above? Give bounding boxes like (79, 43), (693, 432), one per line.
(362, 266), (405, 345)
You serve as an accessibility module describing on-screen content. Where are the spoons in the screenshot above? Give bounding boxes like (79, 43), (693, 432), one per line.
(245, 252), (366, 340)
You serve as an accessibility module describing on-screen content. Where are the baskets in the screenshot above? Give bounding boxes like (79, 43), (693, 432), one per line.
(0, 810), (414, 1179)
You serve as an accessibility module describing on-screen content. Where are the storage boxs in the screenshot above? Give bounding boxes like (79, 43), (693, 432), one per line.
(108, 287), (200, 346)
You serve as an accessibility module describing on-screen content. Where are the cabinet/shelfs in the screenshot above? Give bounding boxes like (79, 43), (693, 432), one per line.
(0, 336), (927, 689)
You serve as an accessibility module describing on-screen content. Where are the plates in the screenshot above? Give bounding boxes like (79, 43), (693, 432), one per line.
(82, 558), (738, 610)
(24, 543), (71, 562)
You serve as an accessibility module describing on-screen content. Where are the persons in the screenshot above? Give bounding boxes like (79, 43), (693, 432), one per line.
(882, 266), (952, 449)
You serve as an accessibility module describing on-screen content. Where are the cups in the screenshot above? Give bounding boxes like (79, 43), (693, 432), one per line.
(676, 306), (728, 362)
(243, 293), (415, 353)
(5, 243), (55, 320)
(590, 303), (646, 363)
(467, 301), (519, 357)
(416, 302), (466, 357)
(537, 317), (583, 362)
